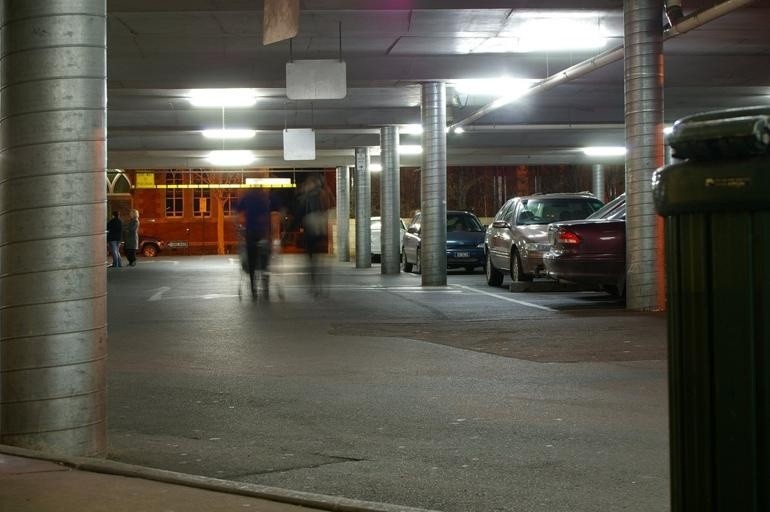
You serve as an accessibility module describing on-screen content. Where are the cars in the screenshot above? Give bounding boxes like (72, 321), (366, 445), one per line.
(540, 190), (626, 288)
(369, 215), (408, 263)
(107, 231), (165, 257)
(401, 208), (488, 274)
(481, 188), (606, 285)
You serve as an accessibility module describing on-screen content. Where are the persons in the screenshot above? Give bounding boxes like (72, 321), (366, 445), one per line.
(124, 209), (140, 267)
(286, 174), (339, 300)
(235, 175), (283, 304)
(108, 211), (123, 268)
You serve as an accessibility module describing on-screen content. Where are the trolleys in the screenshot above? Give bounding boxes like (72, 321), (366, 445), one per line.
(236, 230), (285, 306)
